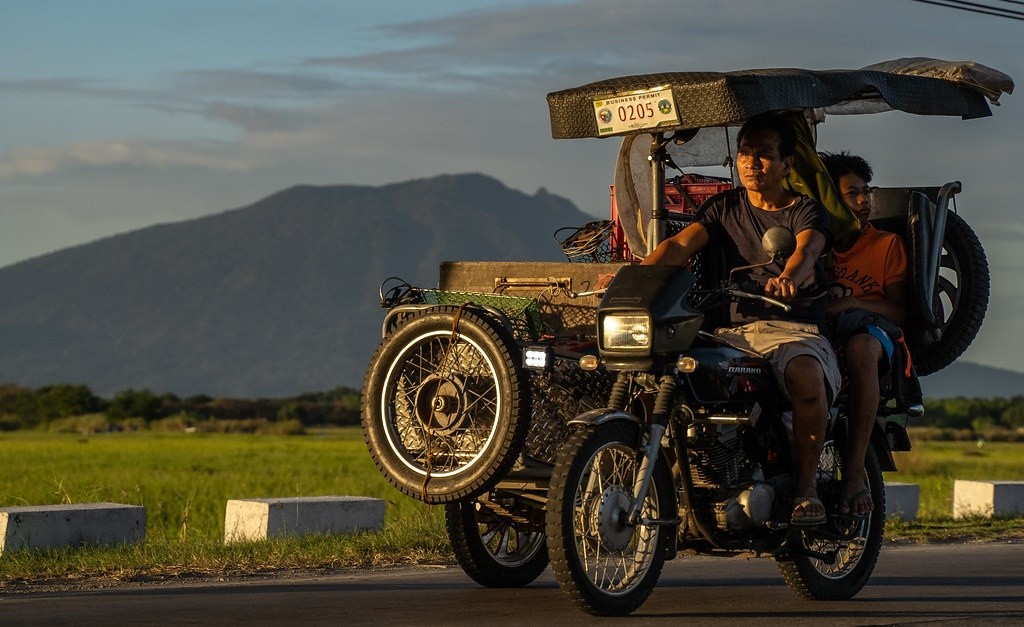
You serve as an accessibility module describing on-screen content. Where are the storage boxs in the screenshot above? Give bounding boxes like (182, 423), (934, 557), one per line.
(438, 259), (639, 334)
(606, 180), (731, 269)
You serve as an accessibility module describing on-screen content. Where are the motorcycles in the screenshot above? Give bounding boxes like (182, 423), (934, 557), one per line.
(362, 57), (1015, 619)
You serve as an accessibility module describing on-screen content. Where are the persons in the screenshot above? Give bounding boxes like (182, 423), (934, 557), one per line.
(595, 115), (840, 527)
(818, 152), (909, 519)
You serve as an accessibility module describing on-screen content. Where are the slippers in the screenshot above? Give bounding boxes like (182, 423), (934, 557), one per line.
(791, 496), (825, 523)
(841, 488), (874, 521)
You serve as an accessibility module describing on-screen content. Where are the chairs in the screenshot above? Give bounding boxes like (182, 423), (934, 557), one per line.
(864, 180), (961, 416)
